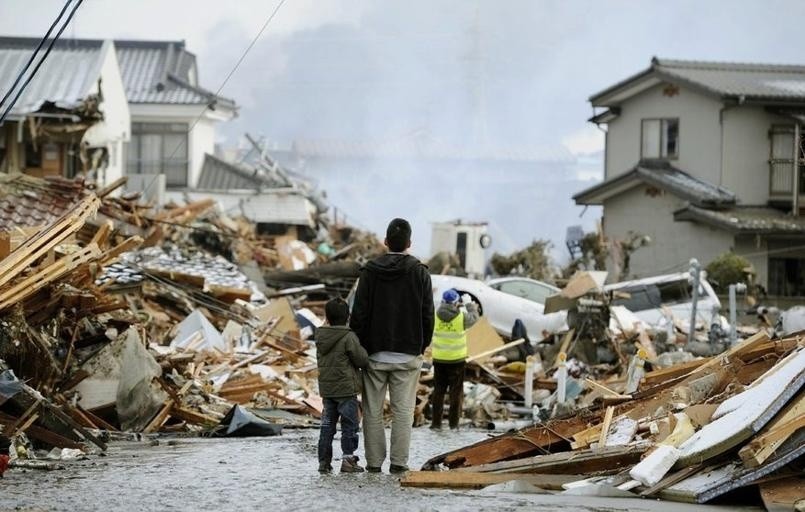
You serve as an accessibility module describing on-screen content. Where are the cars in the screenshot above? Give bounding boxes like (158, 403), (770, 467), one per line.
(429, 272), (720, 346)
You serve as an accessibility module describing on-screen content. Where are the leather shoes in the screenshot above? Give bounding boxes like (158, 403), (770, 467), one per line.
(365, 463), (381, 472)
(389, 464), (409, 473)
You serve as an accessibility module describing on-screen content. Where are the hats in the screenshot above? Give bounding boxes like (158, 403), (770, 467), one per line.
(443, 288), (460, 304)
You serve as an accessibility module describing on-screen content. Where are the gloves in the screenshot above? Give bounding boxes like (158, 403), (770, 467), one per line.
(465, 303), (479, 312)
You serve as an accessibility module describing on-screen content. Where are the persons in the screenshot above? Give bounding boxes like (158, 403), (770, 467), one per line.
(351, 216), (434, 473)
(313, 296), (368, 473)
(427, 286), (479, 429)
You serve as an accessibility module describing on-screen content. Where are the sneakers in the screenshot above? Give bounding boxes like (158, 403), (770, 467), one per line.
(341, 456), (364, 472)
(318, 463), (333, 474)
